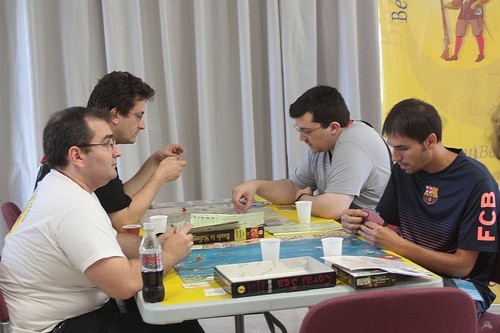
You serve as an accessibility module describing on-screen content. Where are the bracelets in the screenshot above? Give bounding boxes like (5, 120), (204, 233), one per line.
(294, 193), (314, 203)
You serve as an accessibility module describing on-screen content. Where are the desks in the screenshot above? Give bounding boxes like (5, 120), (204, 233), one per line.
(133, 196), (444, 333)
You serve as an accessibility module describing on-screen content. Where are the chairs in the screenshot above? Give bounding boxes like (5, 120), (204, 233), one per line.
(300, 287), (500, 333)
(0, 202), (22, 333)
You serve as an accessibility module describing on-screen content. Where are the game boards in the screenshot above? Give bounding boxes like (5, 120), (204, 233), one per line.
(139, 195), (294, 235)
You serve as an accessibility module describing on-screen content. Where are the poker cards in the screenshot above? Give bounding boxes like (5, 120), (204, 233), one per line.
(174, 218), (187, 234)
(181, 281), (227, 297)
(358, 208), (385, 232)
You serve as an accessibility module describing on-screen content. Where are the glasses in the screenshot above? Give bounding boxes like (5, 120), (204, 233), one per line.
(128, 111), (144, 121)
(292, 121), (334, 136)
(67, 138), (117, 155)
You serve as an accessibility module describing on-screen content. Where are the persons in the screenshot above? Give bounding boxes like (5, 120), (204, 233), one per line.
(341, 98), (500, 321)
(35, 70), (189, 234)
(0, 106), (205, 333)
(232, 86), (396, 222)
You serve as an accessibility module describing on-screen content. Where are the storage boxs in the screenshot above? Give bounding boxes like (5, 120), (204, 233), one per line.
(214, 255), (335, 299)
(331, 263), (396, 289)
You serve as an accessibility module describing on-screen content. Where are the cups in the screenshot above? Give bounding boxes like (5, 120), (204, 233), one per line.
(150, 215), (168, 235)
(321, 237), (343, 264)
(295, 200), (312, 223)
(260, 238), (281, 261)
(122, 224), (141, 235)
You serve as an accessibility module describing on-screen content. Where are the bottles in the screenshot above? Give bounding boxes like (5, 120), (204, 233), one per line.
(139, 222), (165, 303)
